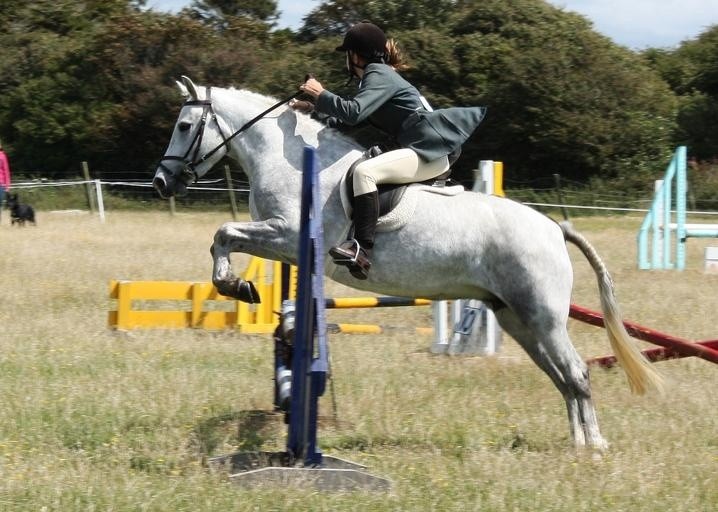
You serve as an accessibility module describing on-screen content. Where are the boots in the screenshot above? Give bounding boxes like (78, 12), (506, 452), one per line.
(328, 190), (380, 280)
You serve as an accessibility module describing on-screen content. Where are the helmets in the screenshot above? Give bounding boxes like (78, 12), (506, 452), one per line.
(336, 20), (386, 58)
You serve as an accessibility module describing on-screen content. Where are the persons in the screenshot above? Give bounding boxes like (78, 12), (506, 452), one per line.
(0, 142), (11, 222)
(289, 18), (488, 279)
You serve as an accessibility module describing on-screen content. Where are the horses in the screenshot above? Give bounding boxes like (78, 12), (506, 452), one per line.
(151, 74), (667, 468)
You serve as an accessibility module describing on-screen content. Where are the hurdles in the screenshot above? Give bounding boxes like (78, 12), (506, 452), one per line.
(198, 147), (390, 489)
